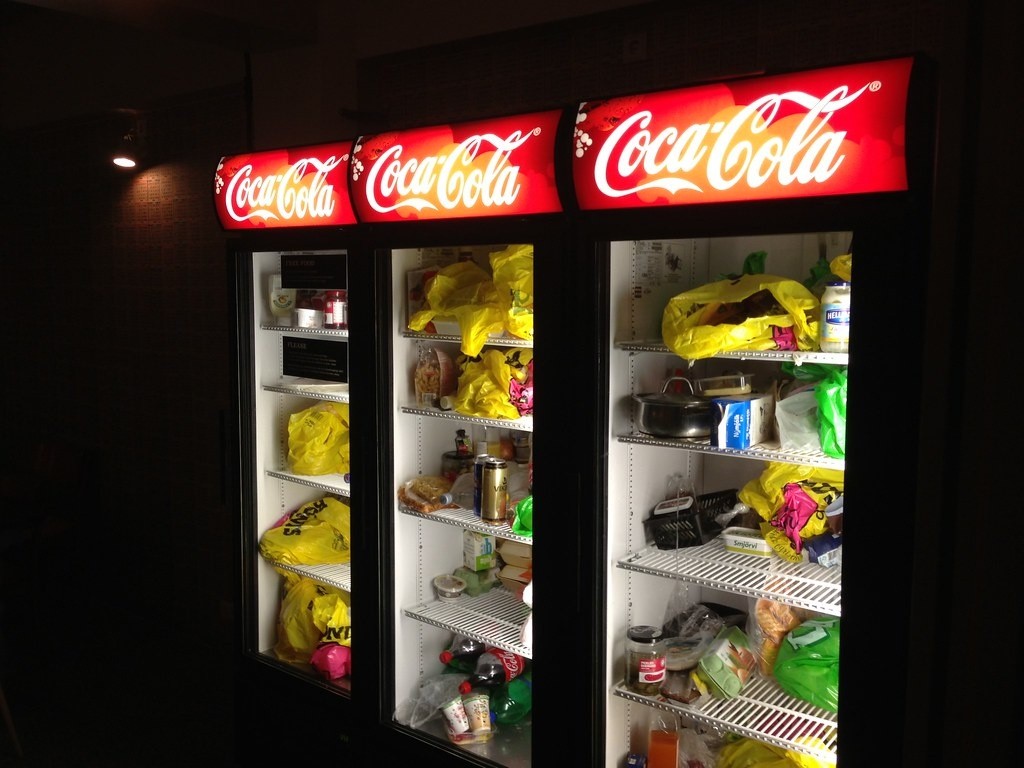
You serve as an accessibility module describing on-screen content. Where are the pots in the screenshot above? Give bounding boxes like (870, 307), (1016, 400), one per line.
(634, 376), (713, 440)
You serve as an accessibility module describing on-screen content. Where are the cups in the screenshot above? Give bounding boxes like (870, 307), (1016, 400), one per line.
(441, 694), (470, 733)
(463, 689), (491, 734)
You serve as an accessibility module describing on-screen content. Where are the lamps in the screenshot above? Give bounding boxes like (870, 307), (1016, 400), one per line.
(110, 109), (147, 171)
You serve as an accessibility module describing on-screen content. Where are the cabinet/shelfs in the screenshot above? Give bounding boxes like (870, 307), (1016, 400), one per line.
(252, 237), (852, 763)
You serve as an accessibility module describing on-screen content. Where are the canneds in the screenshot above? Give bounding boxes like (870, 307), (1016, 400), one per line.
(820, 281), (850, 352)
(321, 290), (348, 330)
(472, 454), (508, 527)
(624, 625), (669, 697)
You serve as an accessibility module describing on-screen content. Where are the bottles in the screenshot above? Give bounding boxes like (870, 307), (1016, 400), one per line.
(268, 274), (296, 326)
(455, 430), (470, 452)
(649, 707), (681, 768)
(471, 424), (502, 460)
(439, 473), (474, 510)
(441, 390), (457, 410)
(440, 630), (531, 724)
